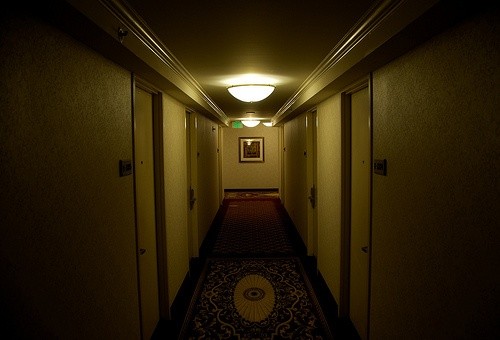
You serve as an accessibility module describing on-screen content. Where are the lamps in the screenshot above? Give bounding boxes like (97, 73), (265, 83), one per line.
(241, 121), (261, 127)
(227, 84), (276, 104)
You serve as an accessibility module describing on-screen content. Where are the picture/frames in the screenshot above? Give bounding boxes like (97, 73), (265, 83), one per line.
(239, 137), (265, 163)
